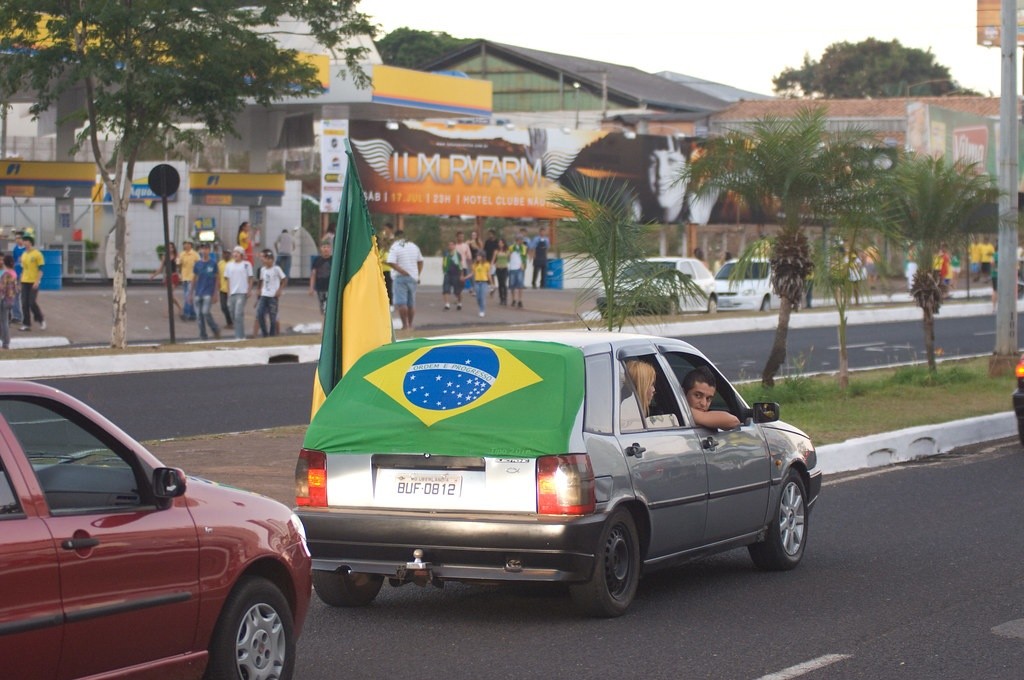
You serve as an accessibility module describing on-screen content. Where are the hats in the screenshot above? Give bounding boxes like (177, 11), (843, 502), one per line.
(234, 246), (245, 255)
(183, 238), (194, 246)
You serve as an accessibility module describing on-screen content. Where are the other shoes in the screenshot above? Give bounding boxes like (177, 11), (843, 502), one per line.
(12, 319), (20, 323)
(517, 302), (523, 308)
(41, 321), (47, 329)
(510, 301), (515, 306)
(224, 323), (232, 328)
(480, 312), (484, 317)
(445, 305), (449, 310)
(457, 305), (461, 310)
(19, 326), (31, 330)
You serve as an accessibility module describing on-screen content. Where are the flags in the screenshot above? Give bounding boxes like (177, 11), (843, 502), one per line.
(310, 152), (394, 427)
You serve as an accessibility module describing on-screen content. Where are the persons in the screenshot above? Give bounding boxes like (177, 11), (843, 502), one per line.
(682, 366), (741, 431)
(694, 248), (733, 275)
(274, 233), (296, 278)
(150, 221), (287, 341)
(625, 359), (656, 416)
(932, 245), (961, 299)
(0, 228), (46, 351)
(968, 237), (995, 282)
(309, 223), (423, 330)
(838, 239), (880, 296)
(442, 228), (551, 317)
(990, 241), (998, 312)
(752, 234), (770, 257)
(905, 243), (921, 294)
(803, 271), (814, 309)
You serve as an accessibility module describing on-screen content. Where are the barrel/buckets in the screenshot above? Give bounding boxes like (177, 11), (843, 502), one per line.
(37, 249), (63, 291)
(543, 257), (563, 289)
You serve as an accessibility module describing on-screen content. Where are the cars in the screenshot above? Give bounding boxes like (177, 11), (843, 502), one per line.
(713, 256), (782, 311)
(0, 378), (313, 680)
(287, 329), (824, 618)
(597, 258), (719, 316)
